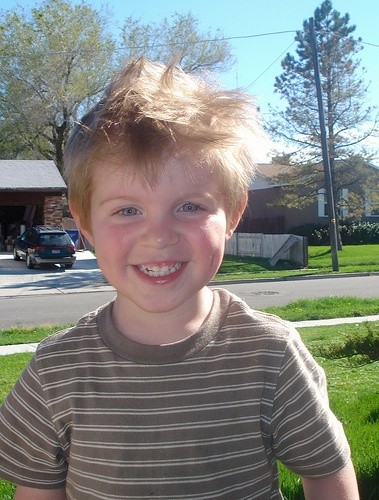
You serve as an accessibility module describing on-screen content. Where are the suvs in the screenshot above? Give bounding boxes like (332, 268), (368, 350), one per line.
(13, 226), (76, 268)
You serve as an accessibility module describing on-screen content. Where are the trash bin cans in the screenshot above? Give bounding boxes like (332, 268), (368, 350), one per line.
(65, 229), (78, 251)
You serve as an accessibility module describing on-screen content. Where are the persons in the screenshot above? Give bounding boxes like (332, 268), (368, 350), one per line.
(0, 53), (361, 500)
